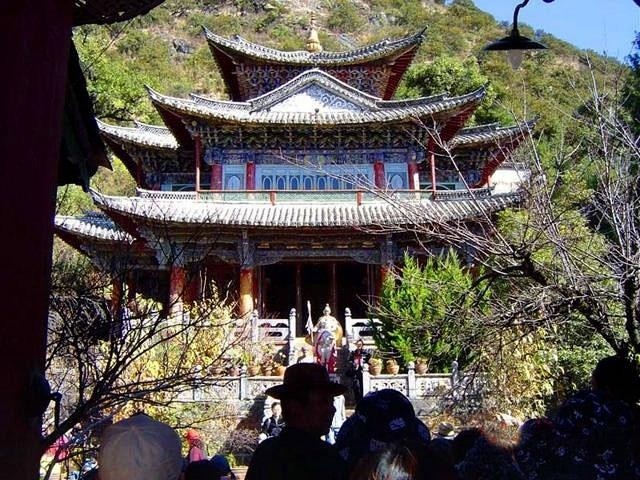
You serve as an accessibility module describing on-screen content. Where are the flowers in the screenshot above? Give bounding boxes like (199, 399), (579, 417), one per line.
(368, 355), (384, 367)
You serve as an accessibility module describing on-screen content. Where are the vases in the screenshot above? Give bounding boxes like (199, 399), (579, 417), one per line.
(416, 359), (428, 374)
(370, 366), (381, 376)
(210, 364), (283, 376)
(387, 365), (400, 374)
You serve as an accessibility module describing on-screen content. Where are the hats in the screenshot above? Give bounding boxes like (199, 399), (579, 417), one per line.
(338, 390), (430, 455)
(98, 415), (183, 480)
(264, 363), (348, 397)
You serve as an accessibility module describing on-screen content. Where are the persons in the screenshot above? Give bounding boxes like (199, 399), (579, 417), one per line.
(42, 356), (640, 479)
(310, 303), (343, 358)
(348, 338), (372, 403)
